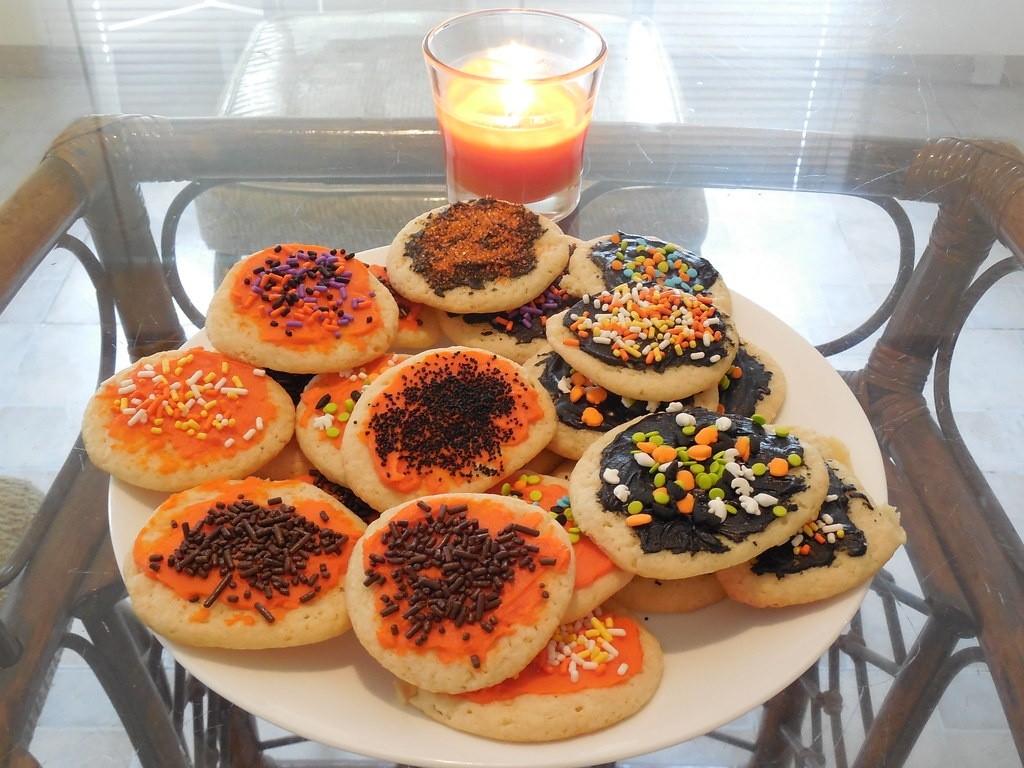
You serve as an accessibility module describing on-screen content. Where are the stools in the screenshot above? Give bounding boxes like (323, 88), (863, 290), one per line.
(193, 7), (709, 295)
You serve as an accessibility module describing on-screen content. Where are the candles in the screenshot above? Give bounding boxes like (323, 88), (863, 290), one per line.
(439, 45), (593, 204)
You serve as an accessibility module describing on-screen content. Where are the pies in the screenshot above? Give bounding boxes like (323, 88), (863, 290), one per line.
(83, 197), (905, 746)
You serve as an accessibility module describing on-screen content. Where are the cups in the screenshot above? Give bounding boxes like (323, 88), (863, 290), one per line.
(424, 9), (607, 223)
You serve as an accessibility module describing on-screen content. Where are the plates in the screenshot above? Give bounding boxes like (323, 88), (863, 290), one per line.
(107, 232), (887, 768)
